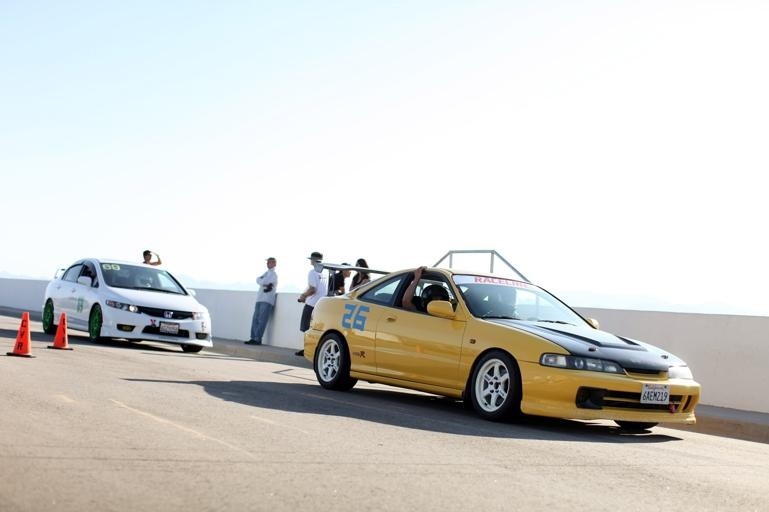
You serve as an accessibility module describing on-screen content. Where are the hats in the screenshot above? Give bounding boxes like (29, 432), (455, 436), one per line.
(307, 252), (322, 260)
(266, 258), (275, 262)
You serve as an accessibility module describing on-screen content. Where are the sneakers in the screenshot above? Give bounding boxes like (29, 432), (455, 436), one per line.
(244, 339), (262, 345)
(295, 350), (304, 356)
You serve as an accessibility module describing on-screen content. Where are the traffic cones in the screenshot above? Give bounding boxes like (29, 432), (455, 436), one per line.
(6, 311), (36, 358)
(47, 312), (74, 351)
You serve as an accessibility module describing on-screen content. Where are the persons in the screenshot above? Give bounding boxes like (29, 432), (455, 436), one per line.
(244, 257), (278, 345)
(295, 252), (329, 356)
(328, 262), (352, 296)
(349, 258), (371, 291)
(142, 250), (162, 266)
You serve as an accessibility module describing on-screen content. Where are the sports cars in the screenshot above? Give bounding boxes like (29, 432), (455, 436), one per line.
(303, 264), (700, 430)
(40, 258), (213, 352)
